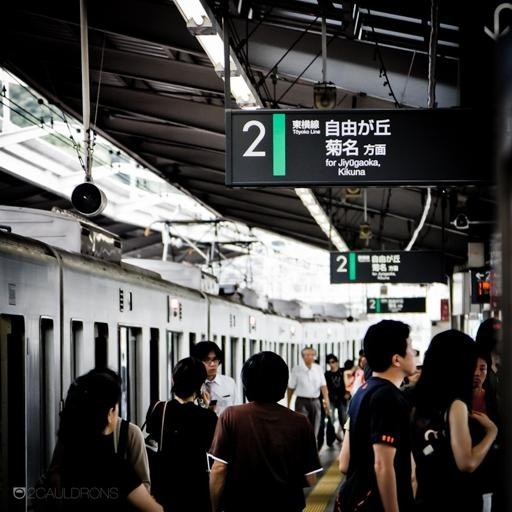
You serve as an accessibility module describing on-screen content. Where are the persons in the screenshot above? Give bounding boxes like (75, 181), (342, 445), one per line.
(35, 317), (512, 512)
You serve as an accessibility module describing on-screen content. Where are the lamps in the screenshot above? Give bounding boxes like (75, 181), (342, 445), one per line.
(358, 188), (373, 242)
(313, 16), (339, 111)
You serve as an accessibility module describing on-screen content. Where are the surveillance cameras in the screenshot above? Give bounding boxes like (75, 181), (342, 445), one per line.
(70, 181), (108, 218)
